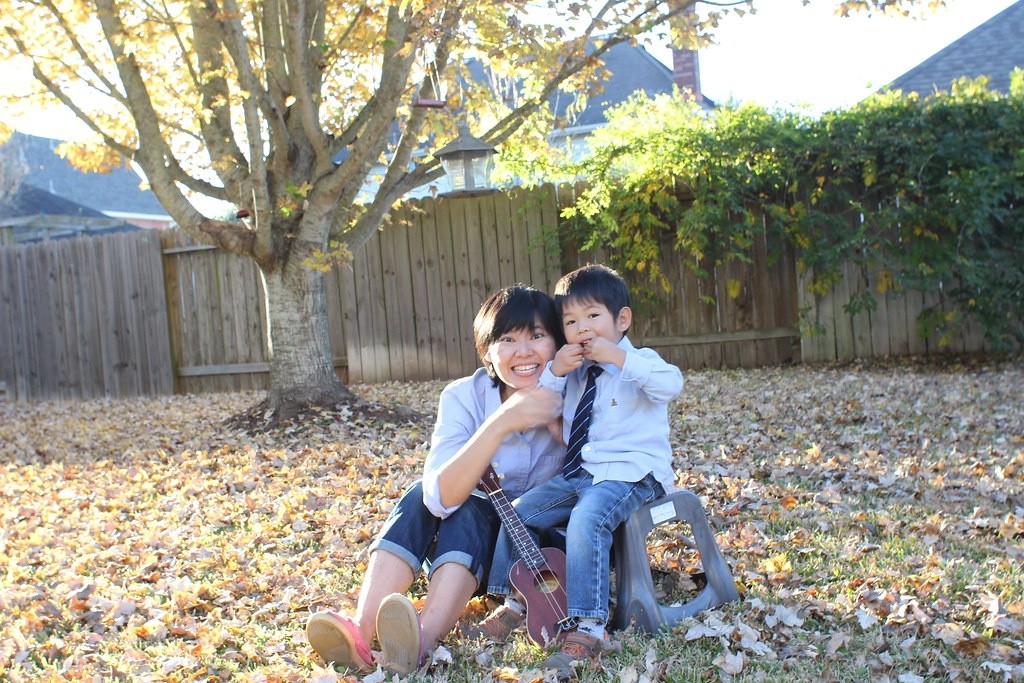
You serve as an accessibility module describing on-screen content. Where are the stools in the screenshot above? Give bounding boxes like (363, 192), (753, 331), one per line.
(548, 488), (738, 635)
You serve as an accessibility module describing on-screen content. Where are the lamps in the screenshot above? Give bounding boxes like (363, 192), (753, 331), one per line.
(435, 122), (502, 199)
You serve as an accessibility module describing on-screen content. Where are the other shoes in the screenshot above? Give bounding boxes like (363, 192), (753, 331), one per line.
(375, 592), (424, 680)
(305, 609), (377, 676)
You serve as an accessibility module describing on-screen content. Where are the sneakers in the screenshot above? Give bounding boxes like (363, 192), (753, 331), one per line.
(541, 628), (609, 679)
(456, 605), (526, 642)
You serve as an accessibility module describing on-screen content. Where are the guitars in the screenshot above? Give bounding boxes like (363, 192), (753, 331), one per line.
(477, 461), (584, 647)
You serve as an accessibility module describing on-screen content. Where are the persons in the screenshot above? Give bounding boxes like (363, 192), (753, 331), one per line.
(456, 264), (683, 681)
(305, 284), (569, 678)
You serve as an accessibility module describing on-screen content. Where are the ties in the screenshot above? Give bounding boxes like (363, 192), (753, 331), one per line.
(562, 365), (605, 480)
